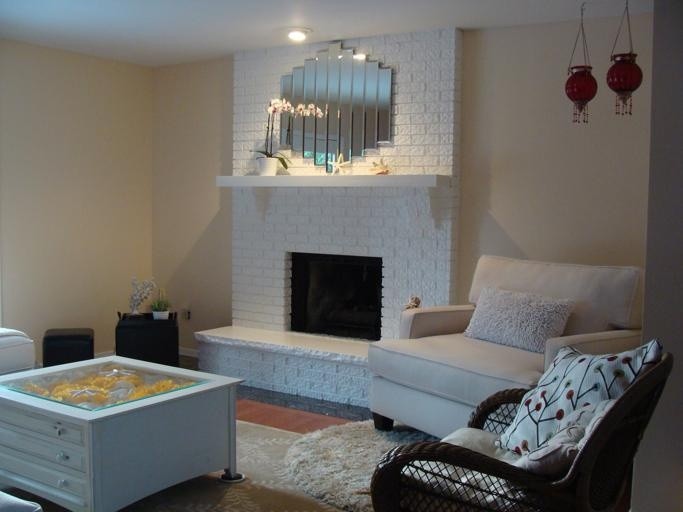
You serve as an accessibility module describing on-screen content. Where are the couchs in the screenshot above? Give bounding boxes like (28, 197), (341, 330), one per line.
(365, 254), (647, 443)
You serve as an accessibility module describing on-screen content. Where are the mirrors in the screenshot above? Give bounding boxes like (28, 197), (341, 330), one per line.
(276, 39), (392, 175)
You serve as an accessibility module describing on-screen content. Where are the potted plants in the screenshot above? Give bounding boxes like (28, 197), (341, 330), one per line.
(148, 299), (173, 320)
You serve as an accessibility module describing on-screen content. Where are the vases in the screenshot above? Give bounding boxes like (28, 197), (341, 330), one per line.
(256, 158), (279, 176)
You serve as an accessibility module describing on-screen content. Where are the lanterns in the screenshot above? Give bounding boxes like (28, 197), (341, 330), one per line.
(564, 64), (598, 125)
(606, 53), (643, 116)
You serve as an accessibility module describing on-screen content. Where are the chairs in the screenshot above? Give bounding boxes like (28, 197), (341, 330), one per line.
(368, 351), (674, 512)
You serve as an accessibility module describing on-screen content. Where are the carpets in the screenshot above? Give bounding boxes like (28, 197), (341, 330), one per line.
(285, 416), (431, 512)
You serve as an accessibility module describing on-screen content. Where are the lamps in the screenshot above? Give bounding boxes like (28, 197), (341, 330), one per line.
(563, 1), (643, 126)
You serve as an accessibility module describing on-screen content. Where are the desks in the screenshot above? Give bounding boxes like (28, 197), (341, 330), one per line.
(112, 312), (179, 365)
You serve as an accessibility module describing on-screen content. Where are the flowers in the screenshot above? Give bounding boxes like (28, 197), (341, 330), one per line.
(128, 276), (156, 309)
(248, 99), (293, 171)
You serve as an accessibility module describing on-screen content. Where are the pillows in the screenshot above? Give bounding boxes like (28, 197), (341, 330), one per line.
(494, 336), (665, 481)
(461, 289), (575, 353)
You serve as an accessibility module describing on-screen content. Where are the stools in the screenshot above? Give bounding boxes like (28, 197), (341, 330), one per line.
(40, 328), (94, 366)
(0, 326), (33, 376)
